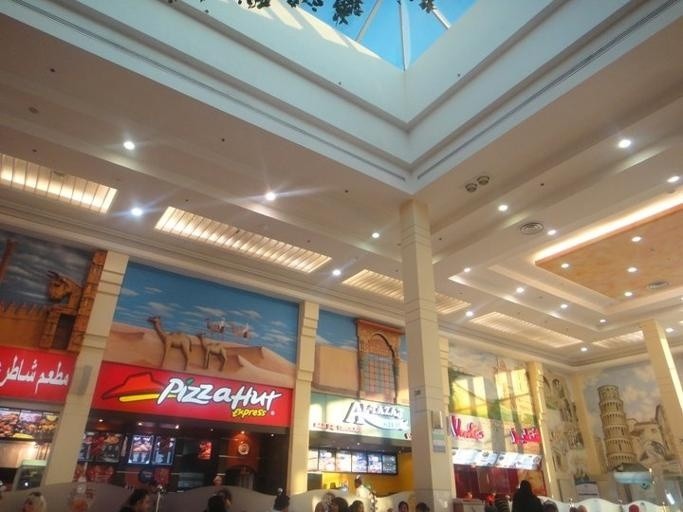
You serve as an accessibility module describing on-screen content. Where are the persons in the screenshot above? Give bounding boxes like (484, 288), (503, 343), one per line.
(20, 491), (47, 512)
(119, 489), (151, 511)
(69, 498), (88, 512)
(628, 503), (640, 512)
(312, 476), (586, 512)
(203, 495), (226, 511)
(268, 495), (290, 512)
(216, 488), (232, 511)
(90, 435), (114, 460)
